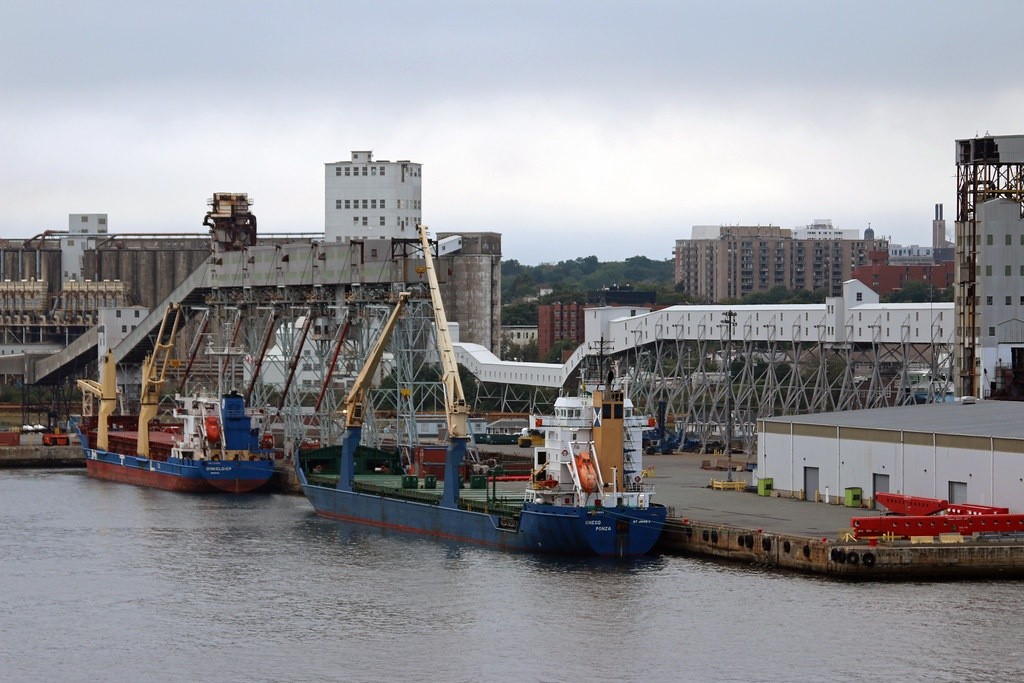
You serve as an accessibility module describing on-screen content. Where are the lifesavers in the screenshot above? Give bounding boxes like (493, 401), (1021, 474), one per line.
(783, 540), (790, 552)
(761, 537), (771, 551)
(830, 548), (846, 564)
(686, 528), (693, 537)
(862, 552), (876, 566)
(703, 530), (709, 541)
(711, 530), (717, 543)
(738, 536), (744, 547)
(803, 544), (810, 557)
(846, 550), (860, 564)
(744, 534), (754, 548)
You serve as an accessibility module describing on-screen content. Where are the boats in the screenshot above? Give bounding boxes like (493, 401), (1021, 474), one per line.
(72, 296), (278, 493)
(290, 219), (668, 560)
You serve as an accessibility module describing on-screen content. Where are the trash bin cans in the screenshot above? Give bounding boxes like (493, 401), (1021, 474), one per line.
(425, 474), (437, 489)
(470, 474), (488, 490)
(457, 474), (465, 489)
(401, 474), (419, 489)
(756, 476), (774, 497)
(844, 485), (863, 508)
